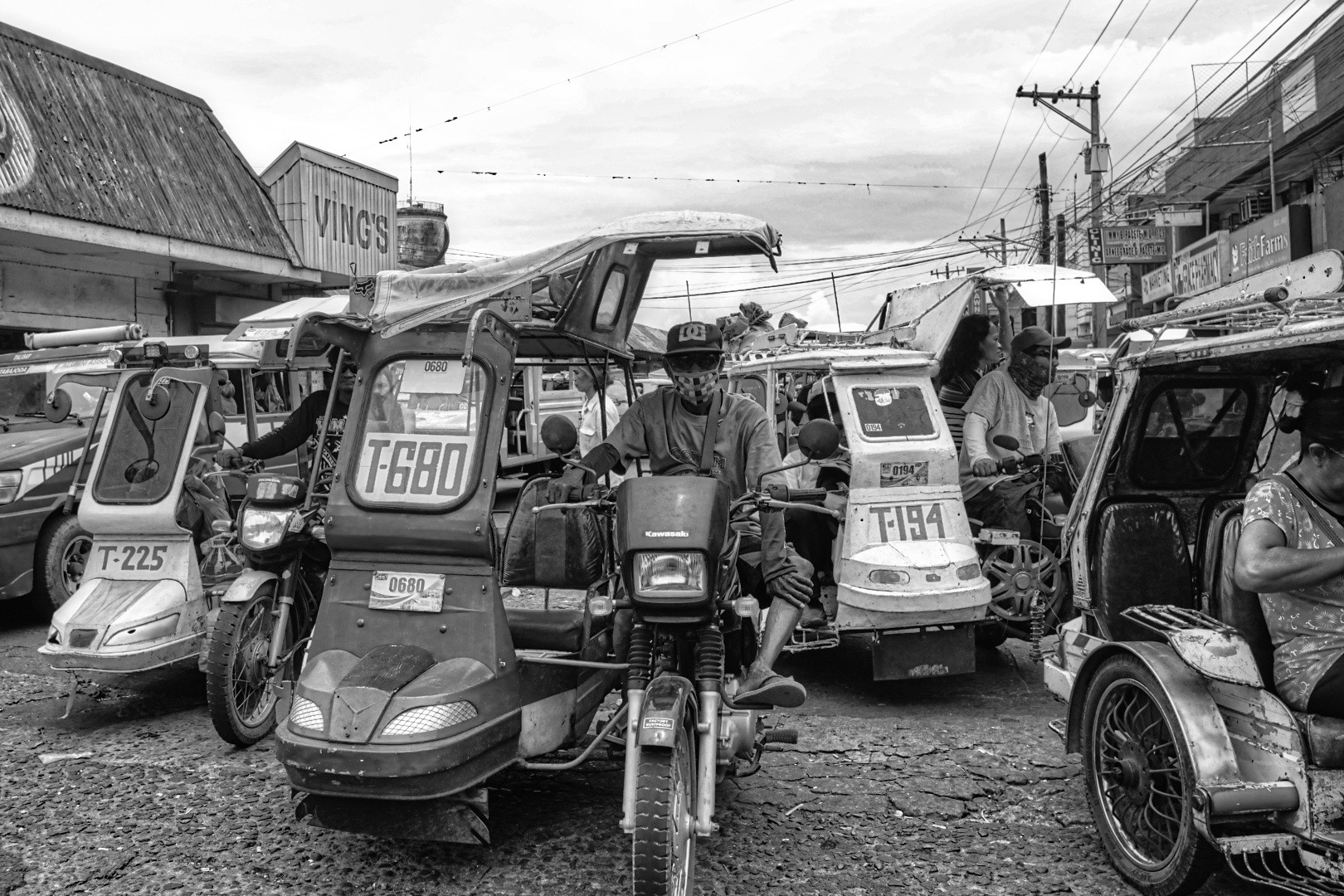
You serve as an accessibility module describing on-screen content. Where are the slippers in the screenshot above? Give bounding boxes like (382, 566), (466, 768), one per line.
(733, 675), (807, 707)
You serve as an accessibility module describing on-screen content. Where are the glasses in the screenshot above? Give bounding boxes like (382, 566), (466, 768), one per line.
(1030, 349), (1059, 359)
(334, 362), (357, 374)
(668, 353), (720, 369)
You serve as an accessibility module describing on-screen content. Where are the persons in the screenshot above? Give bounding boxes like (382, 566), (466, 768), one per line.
(544, 322), (814, 708)
(575, 358), (624, 490)
(937, 284), (1014, 459)
(253, 375), (270, 413)
(370, 368), (417, 434)
(128, 421), (228, 546)
(957, 325), (1062, 539)
(1233, 392), (1344, 720)
(218, 369), (237, 415)
(215, 341), (404, 512)
(780, 391), (852, 608)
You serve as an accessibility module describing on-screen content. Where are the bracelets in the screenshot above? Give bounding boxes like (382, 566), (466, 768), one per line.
(1000, 321), (1012, 330)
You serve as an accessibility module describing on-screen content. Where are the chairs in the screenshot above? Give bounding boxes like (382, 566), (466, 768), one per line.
(489, 473), (604, 652)
(1204, 501), (1245, 615)
(1222, 517), (1344, 764)
(1089, 495), (1197, 637)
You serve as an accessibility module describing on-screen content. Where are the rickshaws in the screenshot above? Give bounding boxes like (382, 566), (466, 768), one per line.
(39, 212), (840, 895)
(633, 264), (1344, 896)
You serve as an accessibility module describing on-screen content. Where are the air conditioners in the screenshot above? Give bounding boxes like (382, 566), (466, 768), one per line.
(1241, 196), (1284, 223)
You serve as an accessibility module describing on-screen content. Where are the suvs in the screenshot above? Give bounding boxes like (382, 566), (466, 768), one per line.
(1, 323), (325, 628)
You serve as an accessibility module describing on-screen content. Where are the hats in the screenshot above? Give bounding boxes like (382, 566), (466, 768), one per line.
(662, 321), (725, 356)
(1010, 326), (1072, 353)
